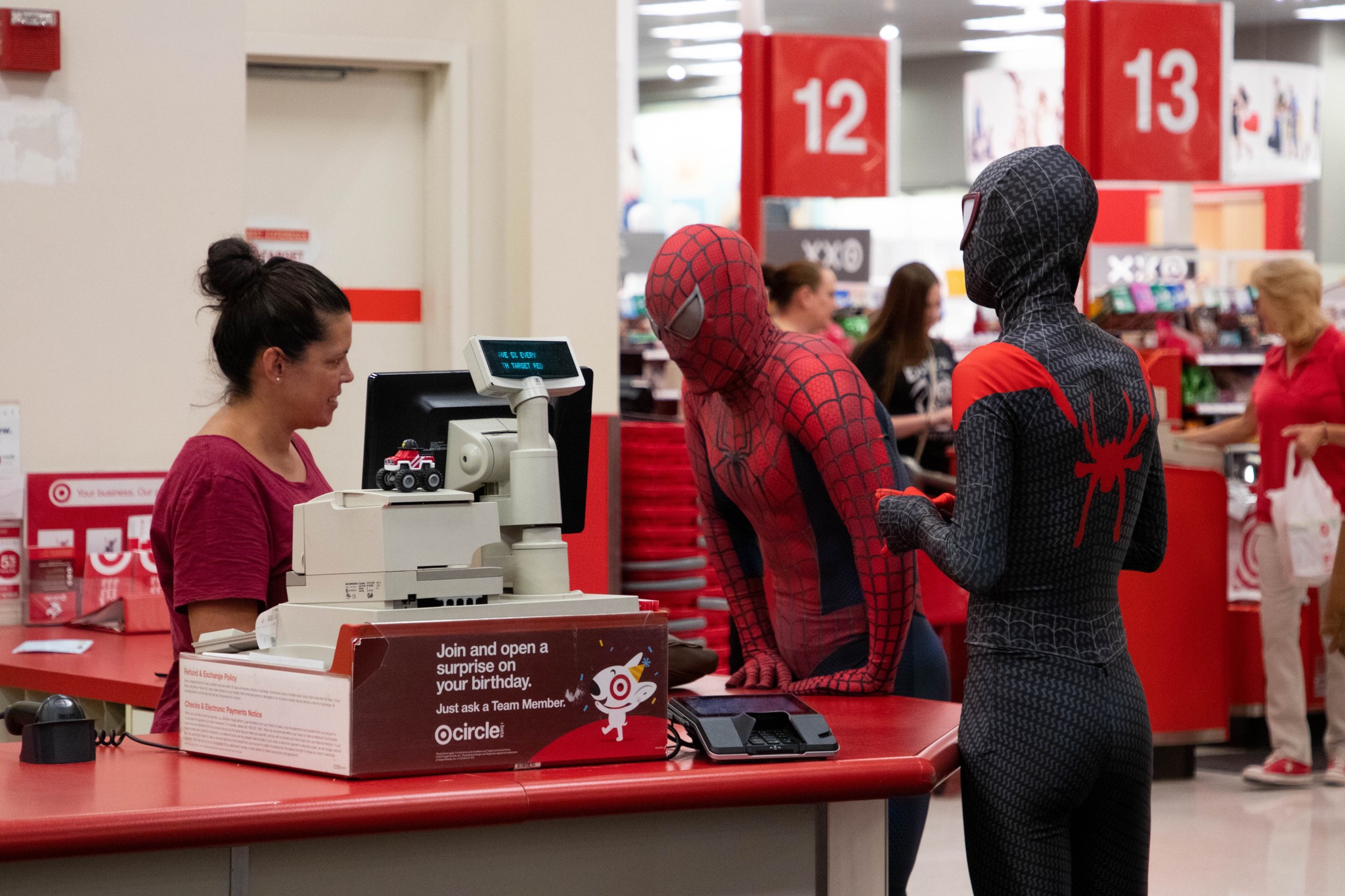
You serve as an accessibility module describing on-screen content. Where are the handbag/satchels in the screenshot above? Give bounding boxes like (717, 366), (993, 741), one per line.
(1265, 441), (1342, 588)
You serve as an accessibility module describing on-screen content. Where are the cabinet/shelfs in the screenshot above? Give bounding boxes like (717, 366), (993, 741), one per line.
(1080, 232), (1345, 476)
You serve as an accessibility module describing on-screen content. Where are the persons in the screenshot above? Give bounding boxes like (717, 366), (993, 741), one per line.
(1175, 257), (1345, 787)
(646, 225), (953, 896)
(755, 254), (842, 337)
(872, 143), (1168, 896)
(149, 238), (357, 736)
(847, 260), (956, 521)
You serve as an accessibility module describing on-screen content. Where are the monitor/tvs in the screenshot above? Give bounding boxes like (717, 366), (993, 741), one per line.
(674, 693), (819, 717)
(361, 366), (594, 535)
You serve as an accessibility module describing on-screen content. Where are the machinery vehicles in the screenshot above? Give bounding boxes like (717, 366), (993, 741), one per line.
(376, 450), (443, 492)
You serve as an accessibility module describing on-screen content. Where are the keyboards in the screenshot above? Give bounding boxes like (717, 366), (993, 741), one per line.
(192, 629), (258, 654)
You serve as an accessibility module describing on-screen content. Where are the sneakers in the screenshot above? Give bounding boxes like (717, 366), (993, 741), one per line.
(1324, 757), (1345, 785)
(1241, 752), (1312, 785)
